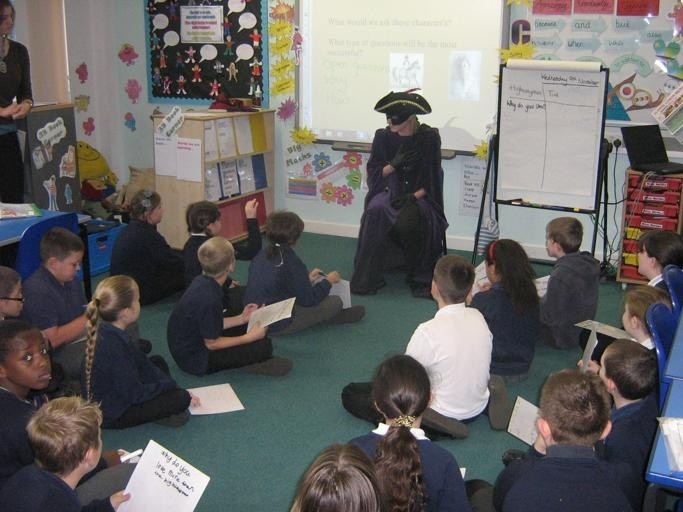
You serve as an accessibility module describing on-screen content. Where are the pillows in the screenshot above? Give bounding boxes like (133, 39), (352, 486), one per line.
(76, 140), (108, 179)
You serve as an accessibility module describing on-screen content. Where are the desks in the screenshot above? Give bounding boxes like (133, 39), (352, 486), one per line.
(659, 309), (682, 384)
(0, 208), (92, 250)
(641, 380), (682, 511)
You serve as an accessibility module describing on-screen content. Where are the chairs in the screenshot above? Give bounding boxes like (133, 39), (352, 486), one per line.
(644, 301), (672, 410)
(16, 212), (78, 281)
(661, 263), (682, 316)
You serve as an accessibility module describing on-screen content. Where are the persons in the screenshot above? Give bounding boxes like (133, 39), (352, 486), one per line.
(350, 88), (449, 299)
(0, 191), (367, 512)
(292, 214), (683, 512)
(0, 0), (33, 271)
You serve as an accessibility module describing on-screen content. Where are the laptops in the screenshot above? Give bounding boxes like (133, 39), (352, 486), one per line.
(621, 124), (683, 175)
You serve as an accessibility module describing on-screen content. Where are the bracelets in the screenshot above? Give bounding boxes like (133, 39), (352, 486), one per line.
(23, 101), (33, 107)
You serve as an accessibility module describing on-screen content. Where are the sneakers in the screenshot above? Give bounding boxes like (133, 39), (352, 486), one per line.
(328, 306), (364, 326)
(487, 378), (512, 430)
(246, 358), (295, 377)
(421, 407), (468, 438)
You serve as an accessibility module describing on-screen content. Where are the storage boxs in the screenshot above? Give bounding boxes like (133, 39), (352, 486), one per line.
(77, 219), (129, 277)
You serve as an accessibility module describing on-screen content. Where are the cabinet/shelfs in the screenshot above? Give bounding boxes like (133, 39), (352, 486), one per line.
(153, 107), (274, 250)
(614, 167), (682, 291)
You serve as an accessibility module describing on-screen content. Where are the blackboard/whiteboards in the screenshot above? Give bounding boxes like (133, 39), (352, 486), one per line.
(498, 63), (610, 214)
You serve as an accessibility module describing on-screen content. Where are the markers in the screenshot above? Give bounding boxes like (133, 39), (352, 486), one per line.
(507, 199), (580, 212)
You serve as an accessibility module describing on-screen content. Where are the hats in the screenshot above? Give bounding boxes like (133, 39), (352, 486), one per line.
(376, 92), (431, 119)
